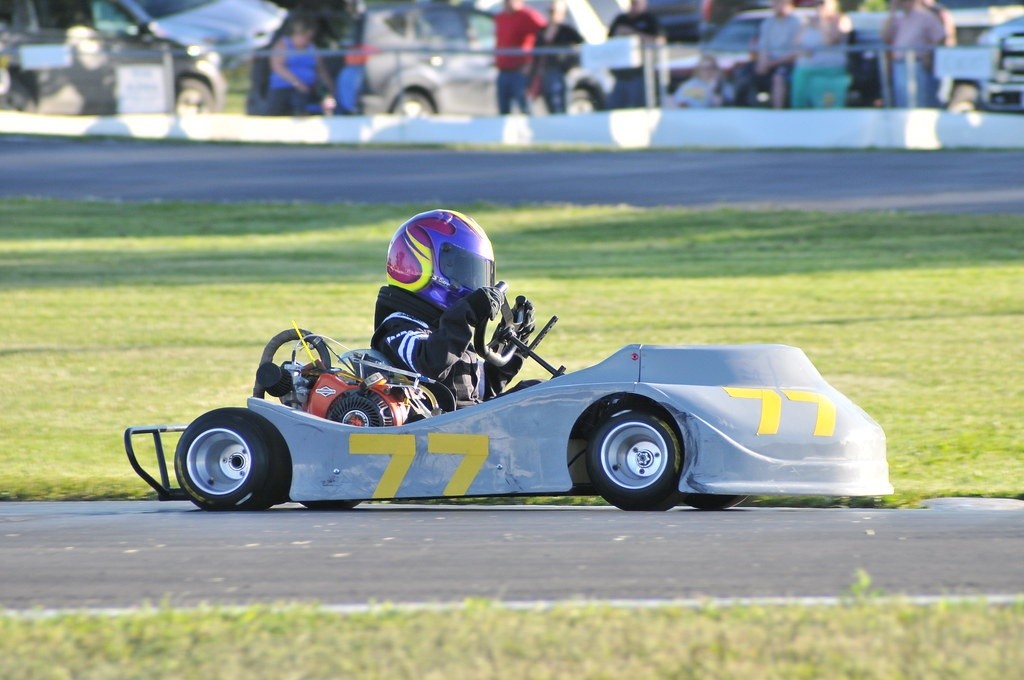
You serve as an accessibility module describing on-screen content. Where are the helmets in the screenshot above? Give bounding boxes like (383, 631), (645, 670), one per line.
(386, 209), (496, 313)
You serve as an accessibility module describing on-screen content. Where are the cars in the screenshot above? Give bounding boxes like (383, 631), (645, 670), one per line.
(655, 8), (880, 107)
(352, 6), (617, 116)
(0, 0), (227, 116)
(136, 0), (291, 71)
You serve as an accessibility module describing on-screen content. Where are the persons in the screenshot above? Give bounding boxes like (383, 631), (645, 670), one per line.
(791, 0), (853, 108)
(251, 13), (335, 115)
(372, 209), (546, 411)
(607, 0), (668, 108)
(881, 0), (956, 109)
(669, 57), (722, 107)
(735, 0), (808, 110)
(496, 0), (547, 114)
(529, 0), (585, 112)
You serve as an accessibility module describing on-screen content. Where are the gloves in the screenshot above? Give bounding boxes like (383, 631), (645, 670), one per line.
(493, 298), (535, 342)
(470, 286), (506, 319)
